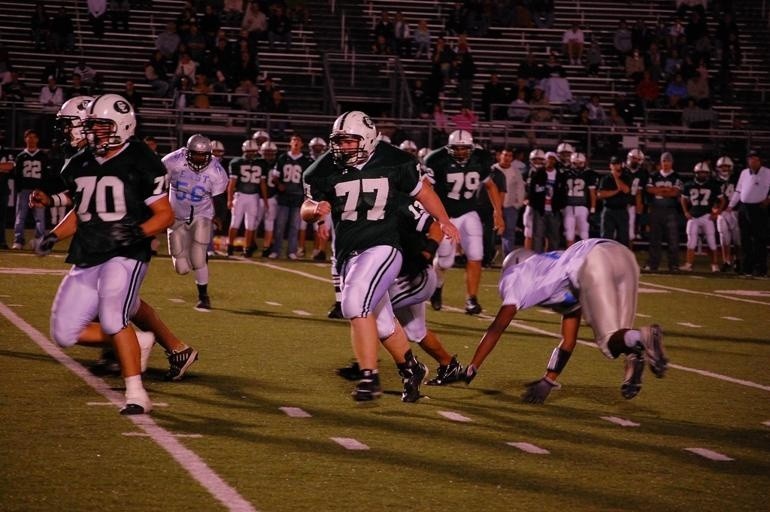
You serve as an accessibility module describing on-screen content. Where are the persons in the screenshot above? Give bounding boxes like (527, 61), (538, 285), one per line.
(529, 151), (565, 252)
(431, 32), (476, 109)
(445, 1), (554, 31)
(87, 0), (131, 38)
(418, 147), (433, 162)
(575, 95), (634, 149)
(144, 135), (160, 155)
(290, 138), (326, 261)
(313, 209), (331, 261)
(74, 60), (97, 85)
(557, 144), (573, 162)
(301, 112), (462, 404)
(715, 158), (742, 271)
(423, 131), (506, 314)
(563, 22), (600, 76)
(465, 239), (668, 402)
(54, 96), (198, 380)
(482, 52), (574, 134)
(640, 152), (682, 273)
(410, 77), (429, 116)
(524, 149), (545, 248)
(12, 130), (51, 250)
(32, 1), (74, 51)
(161, 136), (228, 308)
(63, 72), (87, 100)
(147, 1), (294, 126)
(398, 139), (417, 154)
(253, 130), (268, 146)
(268, 134), (308, 260)
(369, 10), (433, 66)
(207, 140), (225, 258)
(597, 157), (633, 245)
(39, 93), (176, 415)
(40, 75), (64, 105)
(121, 80), (142, 108)
(41, 55), (68, 81)
(563, 152), (596, 248)
(334, 195), (467, 384)
(612, 1), (744, 79)
(1, 68), (24, 107)
(227, 141), (270, 258)
(624, 150), (644, 250)
(725, 149), (770, 278)
(633, 62), (718, 140)
(318, 209), (343, 318)
(682, 161), (725, 271)
(492, 150), (525, 258)
(252, 141), (279, 256)
(0, 145), (16, 249)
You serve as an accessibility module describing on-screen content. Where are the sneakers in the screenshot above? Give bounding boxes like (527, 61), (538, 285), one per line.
(430, 288), (443, 311)
(98, 348), (119, 375)
(12, 243), (22, 249)
(622, 352), (645, 398)
(425, 354), (464, 385)
(351, 368), (382, 401)
(197, 296), (210, 312)
(641, 266), (657, 273)
(336, 362), (360, 380)
(225, 247), (233, 256)
(670, 265), (679, 273)
(288, 253), (297, 260)
(712, 265), (720, 273)
(135, 331), (154, 372)
(312, 248), (320, 257)
(269, 252), (278, 259)
(465, 297), (481, 314)
(640, 324), (667, 378)
(243, 248), (253, 260)
(166, 346), (198, 380)
(297, 248), (305, 256)
(206, 252), (209, 265)
(118, 394), (151, 414)
(679, 263), (692, 271)
(398, 356), (429, 402)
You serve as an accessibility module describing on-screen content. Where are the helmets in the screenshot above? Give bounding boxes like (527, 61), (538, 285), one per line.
(375, 133), (390, 142)
(82, 94), (136, 148)
(330, 110), (376, 166)
(570, 153), (585, 163)
(717, 157), (733, 174)
(185, 134), (212, 170)
(400, 140), (417, 151)
(211, 140), (224, 155)
(529, 149), (545, 159)
(254, 131), (270, 141)
(695, 162), (711, 172)
(243, 139), (258, 151)
(449, 130), (474, 148)
(418, 148), (432, 157)
(501, 247), (535, 271)
(55, 97), (95, 150)
(558, 143), (573, 152)
(259, 141), (277, 151)
(309, 138), (326, 154)
(628, 149), (644, 164)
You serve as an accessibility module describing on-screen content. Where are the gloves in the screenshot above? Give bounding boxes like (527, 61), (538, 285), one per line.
(460, 364), (477, 382)
(106, 222), (144, 250)
(520, 379), (555, 404)
(396, 239), (439, 283)
(33, 233), (55, 256)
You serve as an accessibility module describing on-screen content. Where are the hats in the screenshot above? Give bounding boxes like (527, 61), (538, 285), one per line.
(746, 150), (759, 157)
(610, 156), (621, 163)
